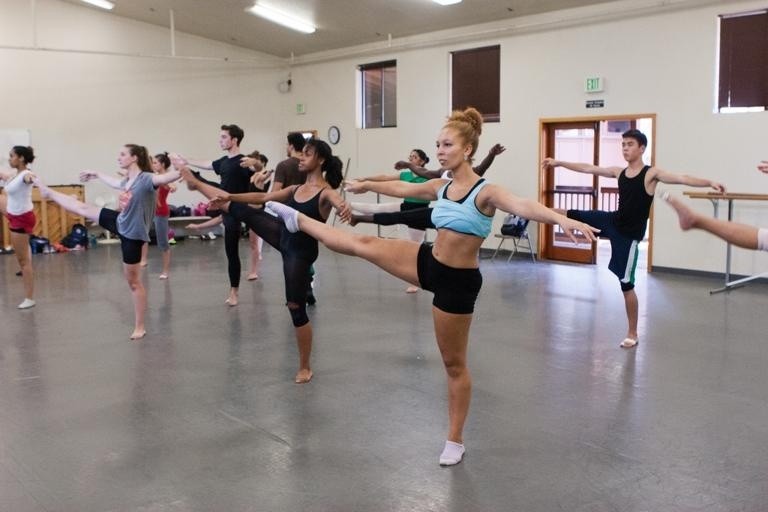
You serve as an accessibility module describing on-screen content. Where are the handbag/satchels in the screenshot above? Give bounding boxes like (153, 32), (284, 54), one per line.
(29, 234), (49, 253)
(500, 213), (527, 237)
(169, 204), (191, 217)
(195, 203), (208, 216)
(62, 224), (89, 248)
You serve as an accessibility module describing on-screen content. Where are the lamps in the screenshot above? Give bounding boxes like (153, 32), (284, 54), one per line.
(244, 2), (316, 39)
(435, 0), (462, 7)
(82, 0), (115, 10)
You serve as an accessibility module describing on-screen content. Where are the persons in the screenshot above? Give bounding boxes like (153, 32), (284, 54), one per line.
(144, 151), (177, 278)
(665, 158), (768, 252)
(183, 130), (351, 382)
(265, 108), (601, 468)
(0, 145), (36, 309)
(32, 144), (183, 340)
(175, 122), (275, 304)
(541, 129), (727, 348)
(333, 142), (504, 293)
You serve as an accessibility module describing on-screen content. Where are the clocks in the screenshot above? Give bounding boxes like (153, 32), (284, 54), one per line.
(327, 125), (339, 144)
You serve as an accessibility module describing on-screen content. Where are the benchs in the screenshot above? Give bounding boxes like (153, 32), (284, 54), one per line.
(99, 215), (227, 246)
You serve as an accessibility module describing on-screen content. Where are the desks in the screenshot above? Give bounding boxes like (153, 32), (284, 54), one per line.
(0, 184), (86, 251)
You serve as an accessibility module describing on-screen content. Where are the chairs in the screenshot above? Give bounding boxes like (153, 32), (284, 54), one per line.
(492, 212), (535, 264)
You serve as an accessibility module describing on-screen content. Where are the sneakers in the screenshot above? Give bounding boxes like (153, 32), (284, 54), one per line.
(188, 232), (217, 240)
